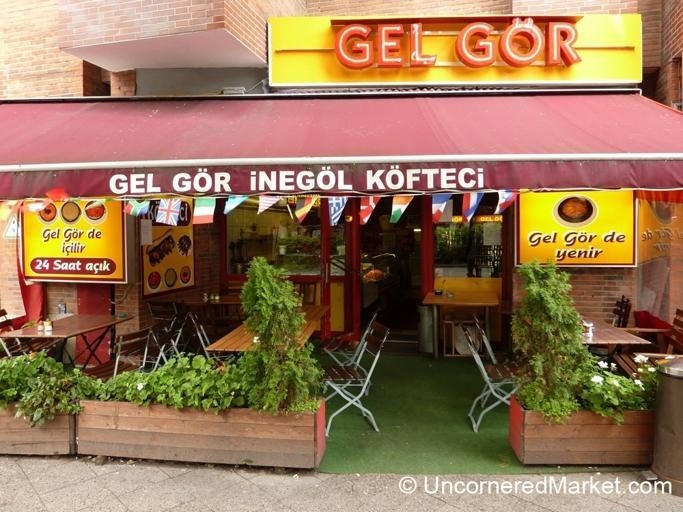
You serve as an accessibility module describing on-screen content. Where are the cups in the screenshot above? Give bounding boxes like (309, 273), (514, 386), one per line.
(583, 321), (595, 337)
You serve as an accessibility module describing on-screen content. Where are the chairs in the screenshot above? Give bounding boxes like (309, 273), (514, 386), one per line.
(617, 294), (683, 379)
(226, 279), (249, 323)
(314, 305), (392, 438)
(82, 299), (245, 380)
(0, 309), (39, 356)
(457, 312), (534, 434)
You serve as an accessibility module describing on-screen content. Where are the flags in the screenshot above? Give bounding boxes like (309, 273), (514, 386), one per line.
(192, 197), (216, 225)
(124, 199), (150, 217)
(431, 193), (452, 222)
(223, 195), (248, 215)
(257, 195), (281, 215)
(294, 194), (318, 225)
(493, 189), (517, 215)
(328, 196), (348, 227)
(390, 194), (414, 224)
(462, 192), (485, 223)
(359, 195), (380, 226)
(156, 198), (182, 227)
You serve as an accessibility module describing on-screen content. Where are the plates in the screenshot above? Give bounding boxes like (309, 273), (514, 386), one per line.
(37, 199), (108, 226)
(176, 243), (191, 257)
(553, 194), (597, 228)
(146, 273), (192, 292)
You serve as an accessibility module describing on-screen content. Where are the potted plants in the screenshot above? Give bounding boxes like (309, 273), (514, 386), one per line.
(1, 349), (77, 456)
(74, 257), (327, 469)
(510, 257), (657, 465)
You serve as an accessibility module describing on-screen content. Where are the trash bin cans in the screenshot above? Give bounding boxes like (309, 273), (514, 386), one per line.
(640, 359), (683, 498)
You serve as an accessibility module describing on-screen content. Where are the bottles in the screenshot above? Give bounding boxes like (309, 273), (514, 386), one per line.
(38, 318), (53, 330)
(202, 291), (220, 300)
